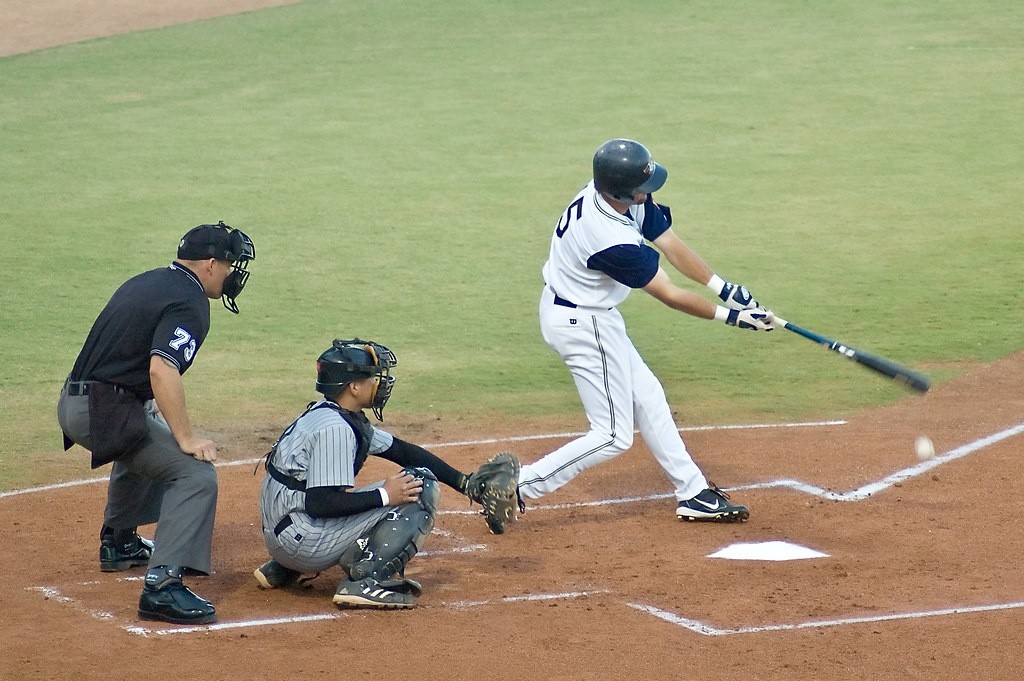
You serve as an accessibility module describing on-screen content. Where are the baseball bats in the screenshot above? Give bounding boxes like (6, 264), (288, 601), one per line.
(772, 316), (933, 397)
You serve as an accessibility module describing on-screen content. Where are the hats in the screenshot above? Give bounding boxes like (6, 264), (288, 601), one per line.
(176, 226), (234, 262)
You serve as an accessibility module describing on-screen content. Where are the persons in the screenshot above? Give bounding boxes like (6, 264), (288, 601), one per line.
(516, 139), (775, 523)
(253, 339), (520, 610)
(58, 225), (255, 625)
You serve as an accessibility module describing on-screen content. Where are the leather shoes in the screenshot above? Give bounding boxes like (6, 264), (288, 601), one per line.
(137, 583), (217, 624)
(101, 535), (157, 572)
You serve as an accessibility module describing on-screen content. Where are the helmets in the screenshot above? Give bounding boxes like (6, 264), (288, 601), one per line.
(592, 138), (669, 202)
(315, 346), (375, 394)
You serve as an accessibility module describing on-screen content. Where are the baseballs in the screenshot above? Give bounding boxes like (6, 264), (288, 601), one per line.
(915, 436), (935, 460)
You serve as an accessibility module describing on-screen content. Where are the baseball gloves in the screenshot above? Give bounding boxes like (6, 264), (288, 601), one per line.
(468, 451), (519, 535)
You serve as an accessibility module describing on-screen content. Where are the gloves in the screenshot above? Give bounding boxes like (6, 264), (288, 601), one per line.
(708, 277), (760, 312)
(714, 303), (777, 335)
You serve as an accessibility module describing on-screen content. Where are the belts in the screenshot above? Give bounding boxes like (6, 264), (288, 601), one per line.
(555, 294), (578, 306)
(62, 374), (99, 400)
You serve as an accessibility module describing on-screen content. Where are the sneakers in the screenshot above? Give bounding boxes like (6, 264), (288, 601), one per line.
(332, 577), (418, 609)
(676, 486), (752, 524)
(254, 555), (297, 589)
